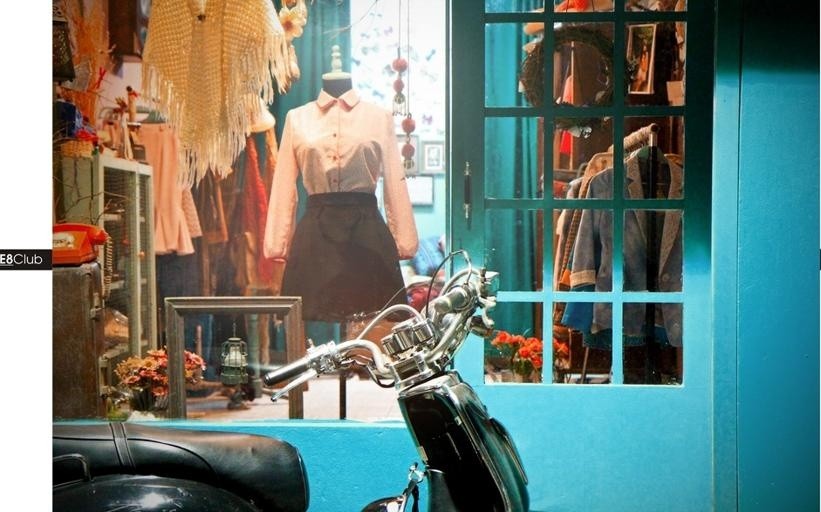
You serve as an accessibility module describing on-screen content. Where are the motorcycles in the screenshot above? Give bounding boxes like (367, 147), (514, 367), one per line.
(53, 244), (532, 512)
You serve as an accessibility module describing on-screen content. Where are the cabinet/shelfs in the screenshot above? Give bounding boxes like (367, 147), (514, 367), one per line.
(54, 149), (158, 422)
(535, 21), (673, 383)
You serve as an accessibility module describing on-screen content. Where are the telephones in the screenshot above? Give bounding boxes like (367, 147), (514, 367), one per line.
(52, 223), (108, 265)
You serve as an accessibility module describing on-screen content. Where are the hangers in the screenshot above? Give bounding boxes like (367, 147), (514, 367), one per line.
(564, 125), (684, 198)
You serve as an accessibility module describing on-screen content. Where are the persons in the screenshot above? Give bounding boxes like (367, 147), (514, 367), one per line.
(262, 44), (421, 324)
(632, 44), (649, 92)
